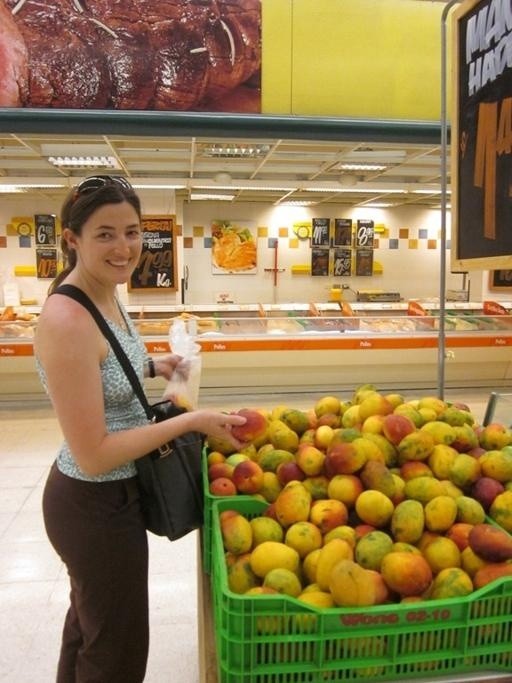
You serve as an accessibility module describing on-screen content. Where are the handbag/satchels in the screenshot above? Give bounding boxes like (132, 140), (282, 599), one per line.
(135, 400), (203, 541)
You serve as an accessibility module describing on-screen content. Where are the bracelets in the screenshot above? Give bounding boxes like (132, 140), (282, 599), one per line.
(147, 355), (156, 379)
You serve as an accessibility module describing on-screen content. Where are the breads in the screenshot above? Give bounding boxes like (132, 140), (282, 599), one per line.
(214, 229), (255, 269)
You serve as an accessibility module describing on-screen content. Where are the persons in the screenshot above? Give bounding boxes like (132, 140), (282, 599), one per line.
(31, 173), (254, 680)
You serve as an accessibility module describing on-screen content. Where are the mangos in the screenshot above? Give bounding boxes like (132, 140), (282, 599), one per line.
(207, 384), (512, 675)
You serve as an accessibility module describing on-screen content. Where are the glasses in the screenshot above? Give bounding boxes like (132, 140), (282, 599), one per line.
(71, 176), (133, 207)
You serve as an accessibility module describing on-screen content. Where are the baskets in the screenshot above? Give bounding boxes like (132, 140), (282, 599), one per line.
(203, 443), (512, 683)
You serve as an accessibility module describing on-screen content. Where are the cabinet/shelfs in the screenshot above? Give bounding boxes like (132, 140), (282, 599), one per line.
(1, 307), (511, 407)
